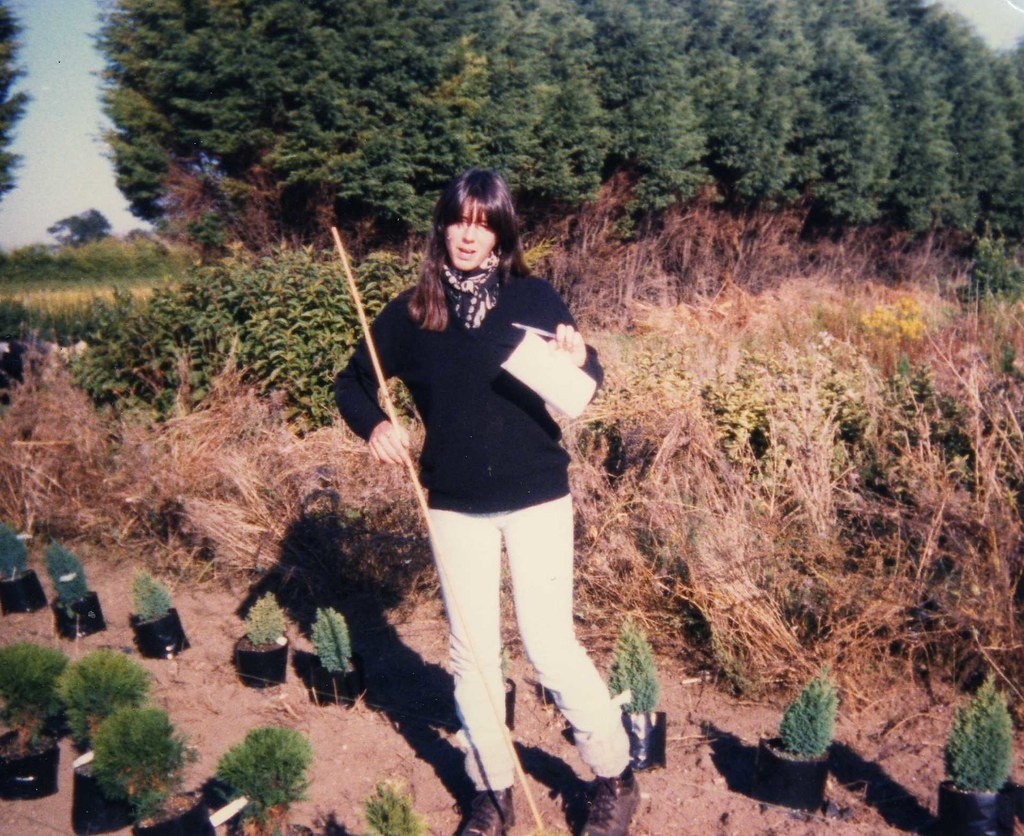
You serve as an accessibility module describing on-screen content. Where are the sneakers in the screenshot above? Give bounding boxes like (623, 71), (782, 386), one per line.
(582, 765), (641, 836)
(462, 787), (516, 836)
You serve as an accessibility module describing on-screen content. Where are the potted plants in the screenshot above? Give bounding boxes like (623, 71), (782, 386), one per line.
(0, 521), (47, 616)
(938, 666), (1015, 836)
(608, 613), (666, 773)
(129, 564), (190, 658)
(0, 641), (315, 836)
(310, 606), (364, 700)
(751, 664), (840, 813)
(235, 590), (289, 688)
(500, 646), (516, 730)
(43, 536), (106, 640)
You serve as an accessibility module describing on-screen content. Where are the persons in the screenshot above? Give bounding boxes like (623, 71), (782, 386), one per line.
(334, 169), (638, 836)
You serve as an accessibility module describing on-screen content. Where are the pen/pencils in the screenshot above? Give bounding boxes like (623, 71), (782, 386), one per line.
(512, 323), (556, 339)
